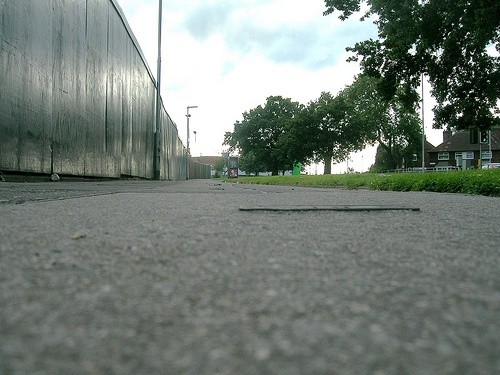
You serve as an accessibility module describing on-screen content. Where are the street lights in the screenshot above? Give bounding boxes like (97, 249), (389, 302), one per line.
(185, 106), (197, 180)
